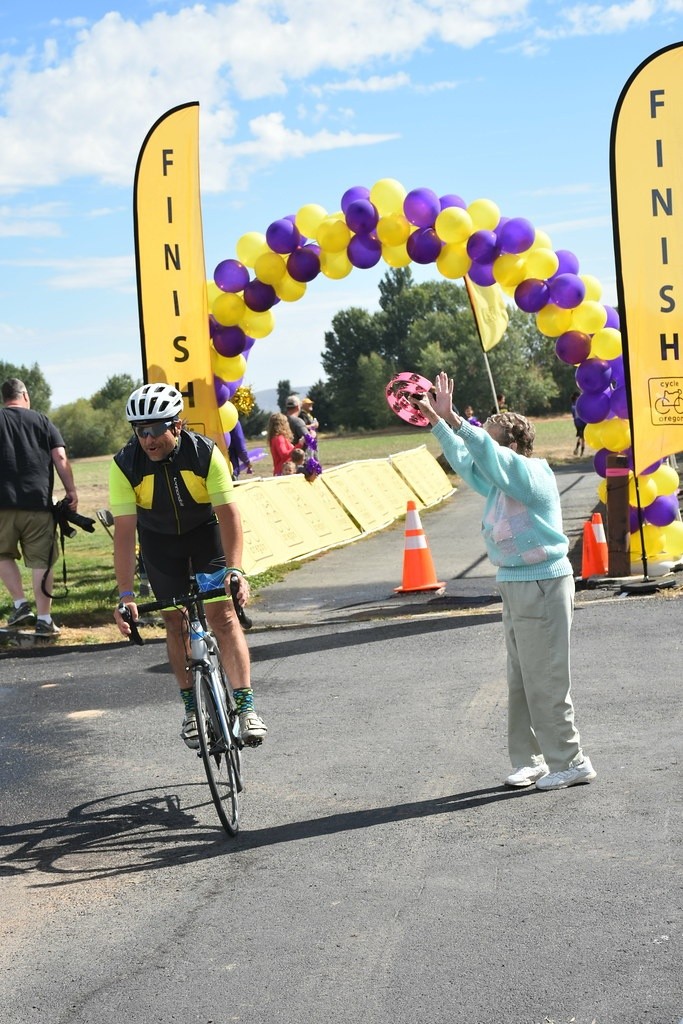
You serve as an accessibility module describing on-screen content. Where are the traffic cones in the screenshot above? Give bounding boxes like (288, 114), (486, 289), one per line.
(393, 501), (447, 593)
(575, 521), (607, 590)
(588, 513), (610, 578)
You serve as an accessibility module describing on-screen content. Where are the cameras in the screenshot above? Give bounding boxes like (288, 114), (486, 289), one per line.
(53, 498), (96, 538)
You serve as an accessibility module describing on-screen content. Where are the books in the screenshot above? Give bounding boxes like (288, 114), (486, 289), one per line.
(212, 377), (239, 448)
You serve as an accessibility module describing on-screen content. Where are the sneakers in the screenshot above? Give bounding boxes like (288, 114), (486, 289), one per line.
(536, 756), (596, 789)
(504, 761), (549, 786)
(34, 619), (61, 636)
(7, 602), (35, 626)
(239, 713), (267, 745)
(180, 715), (200, 749)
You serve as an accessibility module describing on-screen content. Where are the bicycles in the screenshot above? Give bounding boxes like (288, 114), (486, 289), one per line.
(118, 565), (264, 837)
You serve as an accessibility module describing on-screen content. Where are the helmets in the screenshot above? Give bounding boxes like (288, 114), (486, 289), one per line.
(125, 383), (185, 423)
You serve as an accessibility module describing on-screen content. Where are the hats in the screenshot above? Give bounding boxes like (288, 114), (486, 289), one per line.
(302, 398), (314, 404)
(286, 396), (300, 407)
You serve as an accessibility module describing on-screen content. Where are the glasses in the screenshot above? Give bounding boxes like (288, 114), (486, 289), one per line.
(132, 420), (176, 439)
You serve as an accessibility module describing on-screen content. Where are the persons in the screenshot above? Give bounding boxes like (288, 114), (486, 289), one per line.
(490, 394), (509, 414)
(268, 396), (320, 480)
(408, 369), (595, 791)
(463, 405), (477, 425)
(0, 378), (78, 635)
(108, 382), (267, 749)
(571, 390), (589, 456)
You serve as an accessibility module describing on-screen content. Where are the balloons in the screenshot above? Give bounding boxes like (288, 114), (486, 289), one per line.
(213, 214), (324, 313)
(404, 187), (475, 280)
(466, 199), (559, 296)
(597, 464), (683, 562)
(513, 247), (603, 337)
(555, 301), (624, 392)
(293, 179), (414, 280)
(205, 279), (274, 382)
(573, 386), (667, 479)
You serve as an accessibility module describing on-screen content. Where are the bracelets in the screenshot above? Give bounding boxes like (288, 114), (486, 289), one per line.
(119, 592), (133, 600)
(226, 567), (243, 575)
